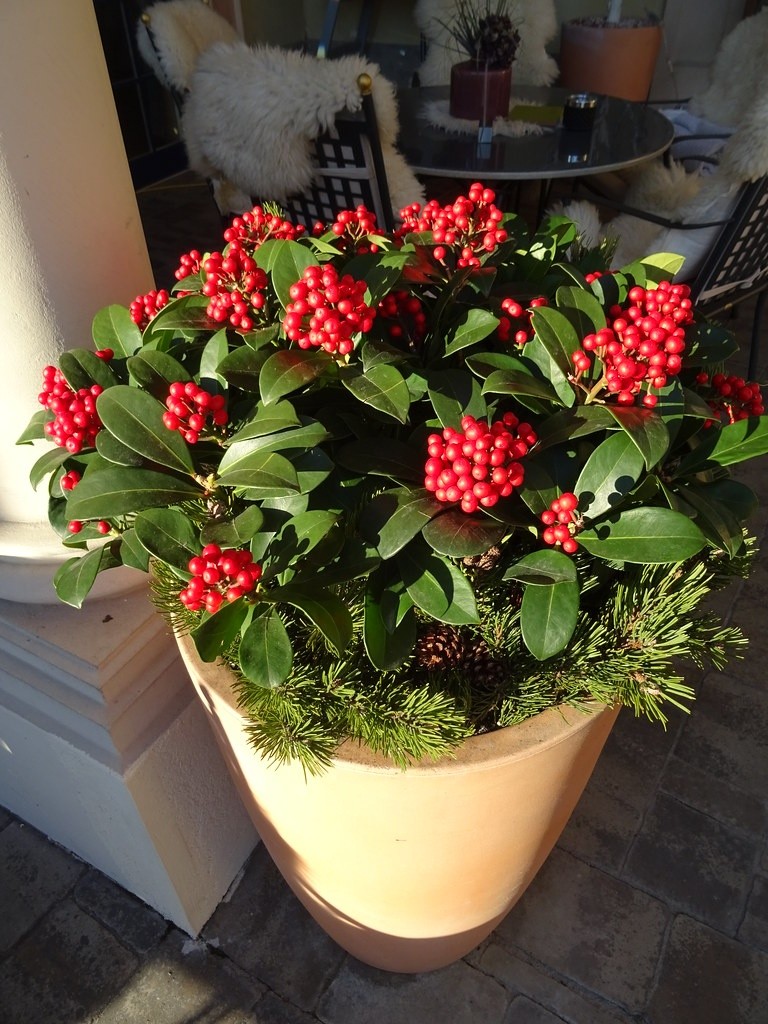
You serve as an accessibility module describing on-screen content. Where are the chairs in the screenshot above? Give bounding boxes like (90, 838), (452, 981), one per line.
(141, 0), (232, 232)
(571, 155), (768, 383)
(251, 73), (395, 236)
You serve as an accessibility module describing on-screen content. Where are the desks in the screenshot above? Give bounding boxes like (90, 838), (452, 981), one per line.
(393, 85), (674, 233)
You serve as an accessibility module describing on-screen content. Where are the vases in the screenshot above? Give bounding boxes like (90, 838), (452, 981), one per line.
(168, 579), (622, 973)
(558, 21), (660, 105)
(450, 61), (512, 121)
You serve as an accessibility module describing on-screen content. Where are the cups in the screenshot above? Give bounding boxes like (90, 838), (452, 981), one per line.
(562, 92), (598, 131)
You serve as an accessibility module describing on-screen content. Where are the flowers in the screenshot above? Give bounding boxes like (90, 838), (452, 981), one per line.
(421, 0), (526, 68)
(16, 184), (768, 785)
